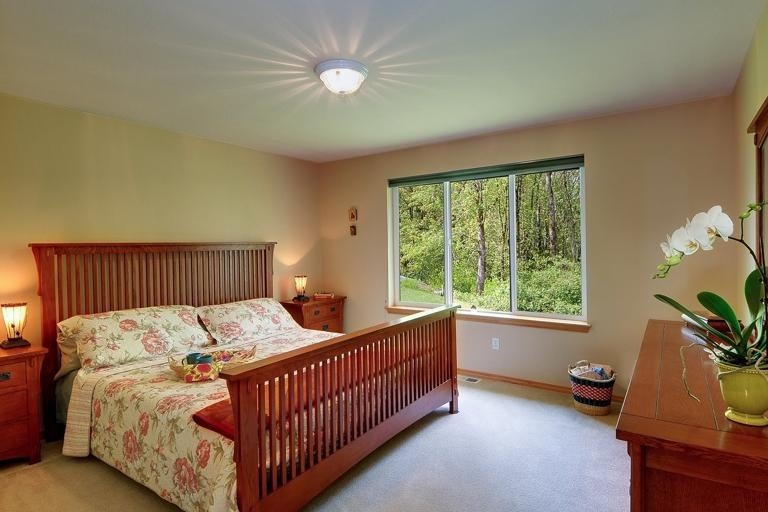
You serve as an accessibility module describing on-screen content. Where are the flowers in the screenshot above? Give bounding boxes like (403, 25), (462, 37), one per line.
(651, 195), (768, 409)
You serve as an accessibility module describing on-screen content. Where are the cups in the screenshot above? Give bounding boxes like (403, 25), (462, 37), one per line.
(197, 354), (213, 366)
(181, 352), (202, 365)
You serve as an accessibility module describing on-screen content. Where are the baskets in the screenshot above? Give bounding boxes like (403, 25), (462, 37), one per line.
(567, 360), (615, 416)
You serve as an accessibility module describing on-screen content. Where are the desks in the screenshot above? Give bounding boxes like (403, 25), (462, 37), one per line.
(608, 318), (767, 512)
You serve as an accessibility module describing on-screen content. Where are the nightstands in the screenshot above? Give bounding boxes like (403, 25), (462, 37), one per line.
(279, 295), (347, 333)
(0, 340), (49, 465)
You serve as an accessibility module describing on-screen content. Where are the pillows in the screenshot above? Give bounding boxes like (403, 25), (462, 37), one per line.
(198, 297), (302, 346)
(57, 304), (213, 374)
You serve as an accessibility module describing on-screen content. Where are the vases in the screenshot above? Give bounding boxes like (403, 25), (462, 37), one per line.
(714, 355), (768, 429)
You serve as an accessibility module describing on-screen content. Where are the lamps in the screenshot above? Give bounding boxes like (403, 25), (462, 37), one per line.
(292, 275), (310, 303)
(0, 301), (32, 348)
(313, 58), (369, 96)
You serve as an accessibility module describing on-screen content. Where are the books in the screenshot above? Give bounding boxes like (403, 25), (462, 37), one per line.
(681, 313), (745, 333)
(312, 290), (335, 302)
(692, 310), (743, 323)
(680, 325), (736, 345)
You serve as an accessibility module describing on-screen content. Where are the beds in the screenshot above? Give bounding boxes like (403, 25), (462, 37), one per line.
(26, 241), (461, 512)
